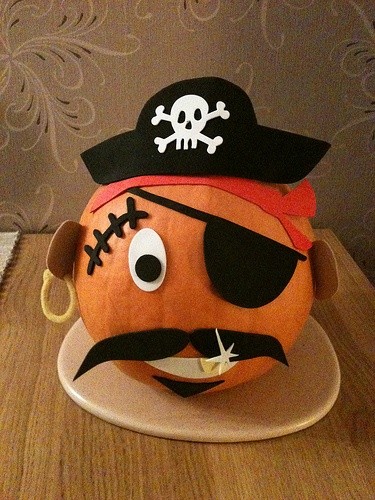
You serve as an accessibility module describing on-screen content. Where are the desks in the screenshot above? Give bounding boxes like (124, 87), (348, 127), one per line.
(0, 230), (375, 499)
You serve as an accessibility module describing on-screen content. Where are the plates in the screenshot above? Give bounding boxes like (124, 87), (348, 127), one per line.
(56, 309), (342, 443)
